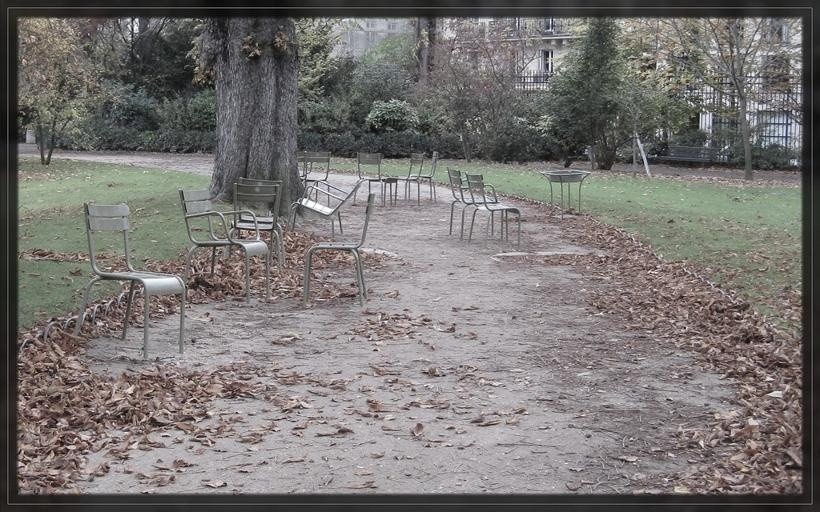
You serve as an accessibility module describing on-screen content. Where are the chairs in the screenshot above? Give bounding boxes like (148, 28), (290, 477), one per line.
(179, 188), (270, 303)
(447, 167), (503, 239)
(303, 193), (375, 307)
(465, 171), (521, 244)
(72, 203), (186, 358)
(224, 151), (438, 274)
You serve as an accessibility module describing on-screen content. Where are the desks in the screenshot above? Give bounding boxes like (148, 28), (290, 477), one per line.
(540, 170), (591, 219)
